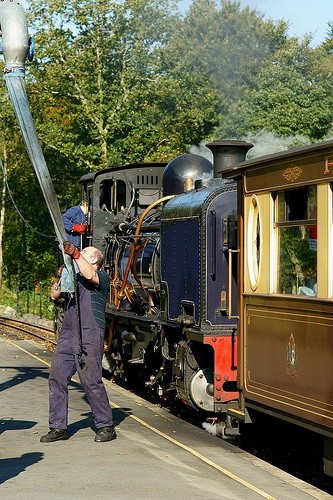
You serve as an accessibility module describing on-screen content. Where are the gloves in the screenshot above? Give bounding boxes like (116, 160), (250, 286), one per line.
(58, 264), (65, 279)
(73, 224), (87, 234)
(63, 241), (80, 259)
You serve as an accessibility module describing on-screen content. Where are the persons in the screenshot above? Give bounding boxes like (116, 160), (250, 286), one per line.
(39, 242), (117, 443)
(60, 197), (93, 296)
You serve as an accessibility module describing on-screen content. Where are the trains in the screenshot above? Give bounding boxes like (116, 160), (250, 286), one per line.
(52, 140), (332, 441)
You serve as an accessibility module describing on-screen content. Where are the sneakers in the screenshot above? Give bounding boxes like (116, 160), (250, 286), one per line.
(40, 429), (69, 442)
(95, 426), (116, 442)
